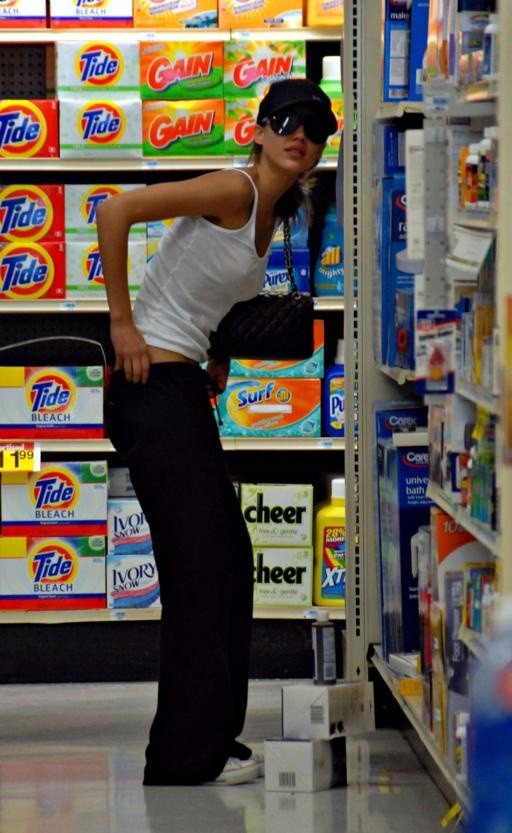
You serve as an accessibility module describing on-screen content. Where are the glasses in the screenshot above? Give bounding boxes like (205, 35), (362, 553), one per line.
(263, 107), (331, 143)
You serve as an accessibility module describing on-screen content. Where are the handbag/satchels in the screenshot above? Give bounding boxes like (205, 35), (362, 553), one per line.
(211, 292), (313, 358)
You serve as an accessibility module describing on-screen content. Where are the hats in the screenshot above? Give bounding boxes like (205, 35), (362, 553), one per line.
(259, 79), (337, 135)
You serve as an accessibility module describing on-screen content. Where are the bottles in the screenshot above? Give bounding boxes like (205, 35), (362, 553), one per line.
(463, 125), (497, 213)
(311, 609), (338, 686)
(480, 13), (500, 81)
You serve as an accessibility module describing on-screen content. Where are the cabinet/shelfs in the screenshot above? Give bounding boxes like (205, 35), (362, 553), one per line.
(344, 0), (511, 833)
(3, 0), (342, 623)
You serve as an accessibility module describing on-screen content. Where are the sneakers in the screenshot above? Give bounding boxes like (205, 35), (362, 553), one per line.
(209, 744), (266, 788)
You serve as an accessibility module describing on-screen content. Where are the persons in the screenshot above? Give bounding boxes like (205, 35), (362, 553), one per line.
(94, 78), (339, 788)
